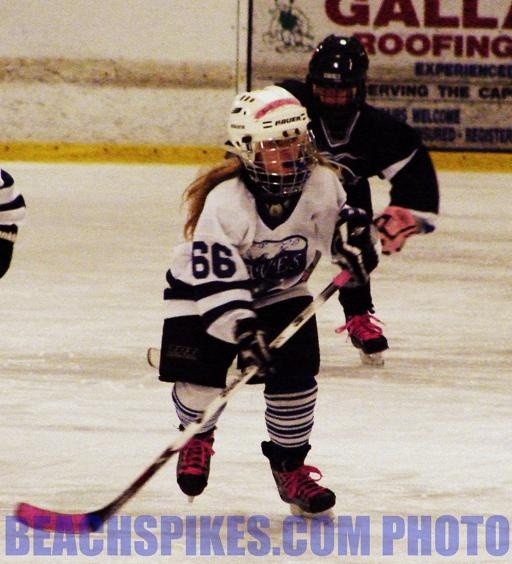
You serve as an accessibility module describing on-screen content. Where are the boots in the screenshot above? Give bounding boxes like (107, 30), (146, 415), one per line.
(261, 441), (337, 514)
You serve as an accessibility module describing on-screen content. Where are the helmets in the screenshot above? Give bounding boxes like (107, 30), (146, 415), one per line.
(226, 84), (316, 197)
(304, 33), (369, 116)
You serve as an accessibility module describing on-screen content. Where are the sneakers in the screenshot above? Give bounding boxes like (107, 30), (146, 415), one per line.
(342, 303), (388, 355)
(176, 424), (216, 496)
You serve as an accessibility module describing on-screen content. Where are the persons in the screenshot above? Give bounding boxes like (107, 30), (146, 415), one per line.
(160, 85), (385, 512)
(264, 29), (443, 360)
(0, 168), (28, 276)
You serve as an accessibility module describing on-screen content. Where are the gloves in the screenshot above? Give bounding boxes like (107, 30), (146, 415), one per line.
(374, 206), (418, 257)
(229, 316), (287, 393)
(327, 205), (379, 284)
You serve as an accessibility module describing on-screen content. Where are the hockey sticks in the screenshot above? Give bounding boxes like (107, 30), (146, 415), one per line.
(15, 269), (352, 534)
